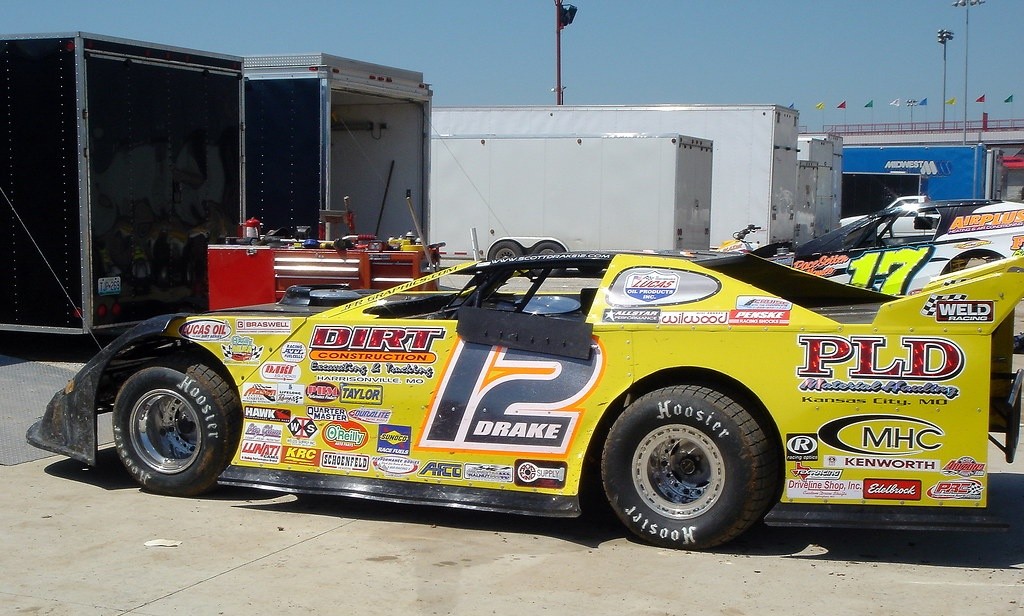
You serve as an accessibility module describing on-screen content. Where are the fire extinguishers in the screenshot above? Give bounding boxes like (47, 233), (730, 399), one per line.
(245, 213), (262, 238)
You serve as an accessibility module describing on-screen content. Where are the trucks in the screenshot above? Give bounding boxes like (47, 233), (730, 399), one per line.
(429, 134), (713, 277)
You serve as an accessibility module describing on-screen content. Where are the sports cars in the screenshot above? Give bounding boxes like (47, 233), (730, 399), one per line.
(29, 249), (1023, 553)
(723, 200), (1024, 298)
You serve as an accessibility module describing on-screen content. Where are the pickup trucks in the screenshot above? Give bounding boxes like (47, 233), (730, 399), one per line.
(840, 195), (943, 236)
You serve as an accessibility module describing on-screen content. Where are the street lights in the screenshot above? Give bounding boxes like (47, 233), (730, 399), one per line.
(905, 99), (918, 135)
(937, 29), (954, 133)
(952, 0), (987, 146)
